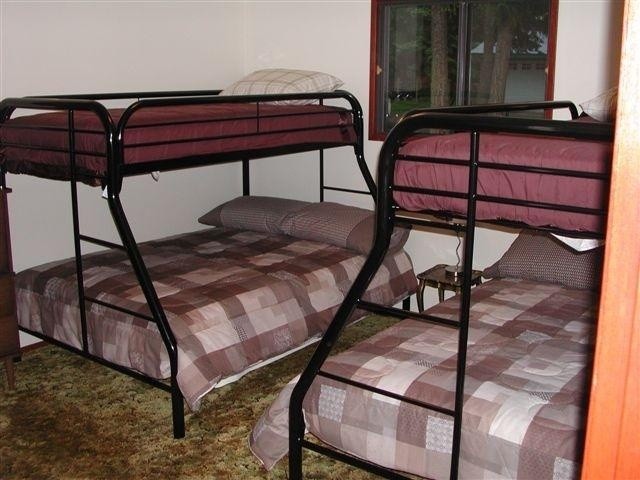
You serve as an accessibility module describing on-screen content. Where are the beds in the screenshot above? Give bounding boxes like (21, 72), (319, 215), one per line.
(375, 96), (616, 245)
(15, 228), (415, 440)
(252, 276), (604, 479)
(2, 90), (358, 186)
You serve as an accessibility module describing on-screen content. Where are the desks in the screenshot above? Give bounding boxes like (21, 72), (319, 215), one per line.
(415, 264), (485, 313)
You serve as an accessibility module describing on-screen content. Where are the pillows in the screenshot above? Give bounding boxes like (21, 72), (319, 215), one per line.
(482, 229), (604, 290)
(579, 90), (616, 124)
(218, 69), (344, 107)
(197, 195), (311, 233)
(280, 201), (412, 259)
